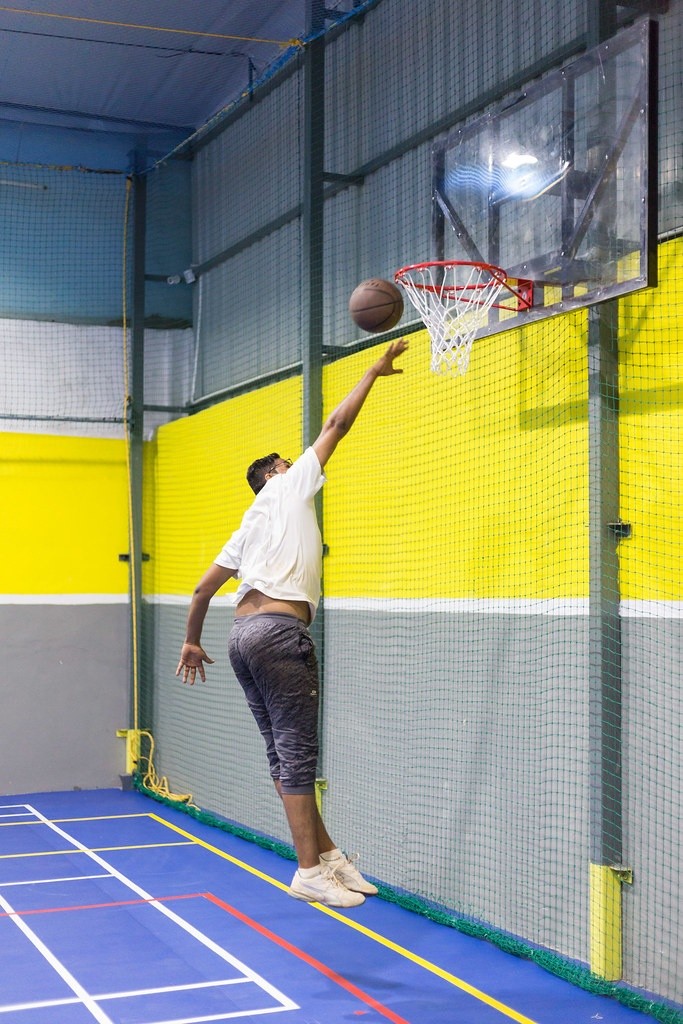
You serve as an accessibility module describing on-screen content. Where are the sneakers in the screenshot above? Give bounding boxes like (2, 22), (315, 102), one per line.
(320, 851), (379, 894)
(289, 865), (366, 908)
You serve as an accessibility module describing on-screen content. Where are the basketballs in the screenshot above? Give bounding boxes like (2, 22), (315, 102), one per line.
(348, 278), (405, 335)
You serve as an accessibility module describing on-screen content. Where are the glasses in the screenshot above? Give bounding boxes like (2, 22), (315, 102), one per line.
(268, 458), (293, 474)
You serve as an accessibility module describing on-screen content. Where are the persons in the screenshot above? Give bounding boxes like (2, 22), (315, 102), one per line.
(176, 338), (409, 908)
